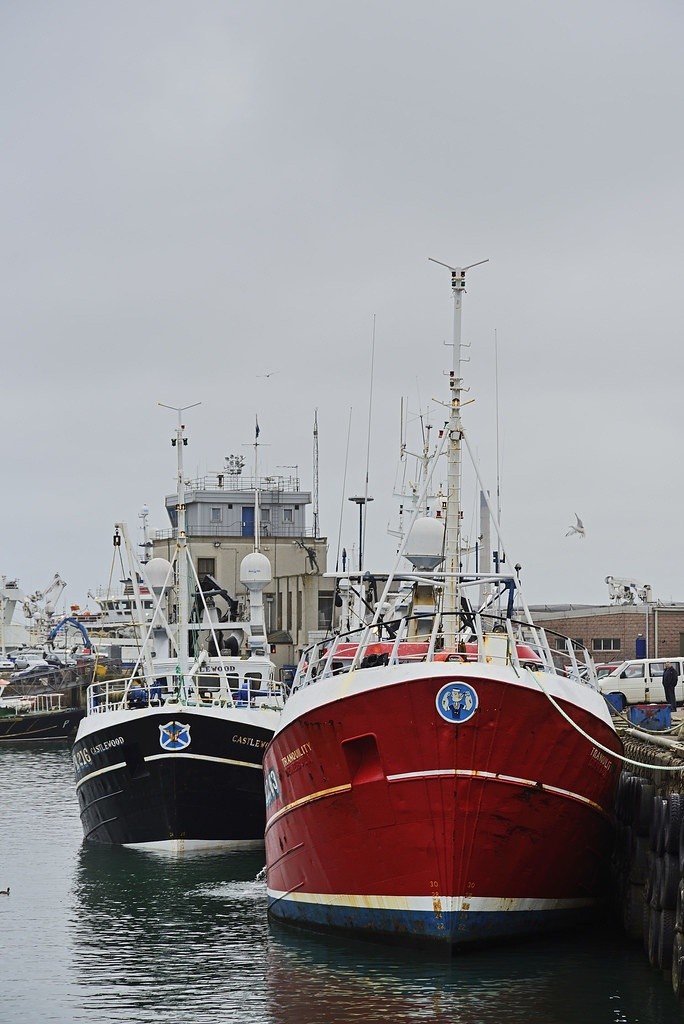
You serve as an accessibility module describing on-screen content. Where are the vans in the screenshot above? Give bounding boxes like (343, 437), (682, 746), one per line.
(596, 656), (684, 707)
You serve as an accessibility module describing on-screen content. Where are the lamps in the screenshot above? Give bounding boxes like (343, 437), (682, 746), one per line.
(151, 652), (156, 658)
(492, 622), (505, 633)
(214, 542), (221, 547)
(638, 633), (643, 637)
(221, 649), (231, 656)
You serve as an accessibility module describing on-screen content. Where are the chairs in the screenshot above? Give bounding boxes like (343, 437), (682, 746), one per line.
(600, 692), (622, 716)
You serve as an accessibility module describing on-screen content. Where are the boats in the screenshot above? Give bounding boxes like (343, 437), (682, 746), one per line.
(0, 396), (307, 857)
(258, 254), (626, 949)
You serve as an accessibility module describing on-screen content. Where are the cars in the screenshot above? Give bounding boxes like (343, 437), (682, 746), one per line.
(563, 656), (660, 686)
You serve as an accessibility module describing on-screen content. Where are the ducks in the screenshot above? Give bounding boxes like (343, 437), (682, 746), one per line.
(0, 887), (11, 894)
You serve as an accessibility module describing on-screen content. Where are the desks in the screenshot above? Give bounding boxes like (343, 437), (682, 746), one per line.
(625, 703), (673, 735)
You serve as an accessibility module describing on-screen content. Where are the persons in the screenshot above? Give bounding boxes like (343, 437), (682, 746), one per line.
(130, 671), (141, 686)
(662, 661), (678, 712)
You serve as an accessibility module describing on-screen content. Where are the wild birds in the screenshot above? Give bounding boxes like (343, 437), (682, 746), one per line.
(565, 512), (586, 540)
